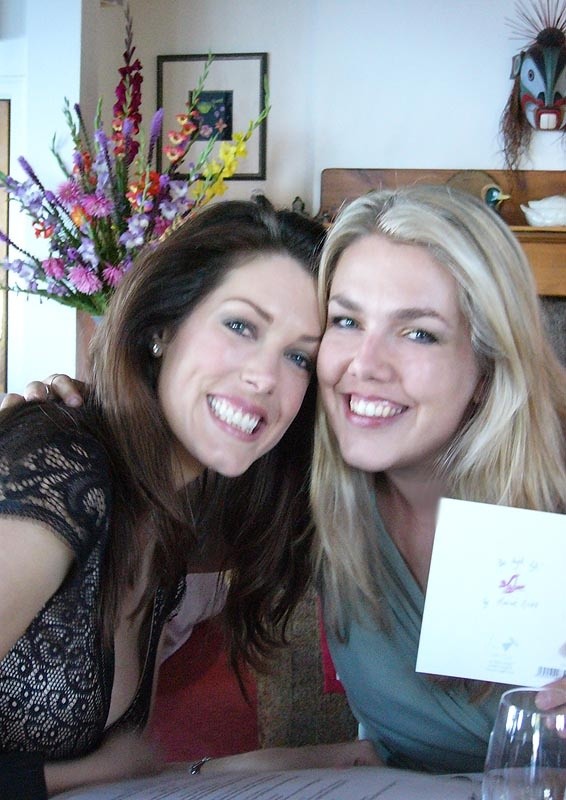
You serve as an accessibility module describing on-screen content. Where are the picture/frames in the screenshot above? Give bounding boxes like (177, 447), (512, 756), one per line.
(156, 54), (267, 179)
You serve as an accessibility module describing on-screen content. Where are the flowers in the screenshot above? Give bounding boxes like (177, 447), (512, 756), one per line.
(1, 0), (273, 317)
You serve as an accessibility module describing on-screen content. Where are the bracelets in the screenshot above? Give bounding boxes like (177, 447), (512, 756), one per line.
(190, 756), (211, 774)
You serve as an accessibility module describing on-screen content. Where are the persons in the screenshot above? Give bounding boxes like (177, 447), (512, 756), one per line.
(0, 170), (566, 800)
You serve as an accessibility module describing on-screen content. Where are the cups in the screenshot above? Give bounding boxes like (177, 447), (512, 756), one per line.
(481, 689), (566, 800)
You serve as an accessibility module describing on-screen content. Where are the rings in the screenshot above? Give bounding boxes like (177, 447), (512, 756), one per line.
(49, 374), (74, 396)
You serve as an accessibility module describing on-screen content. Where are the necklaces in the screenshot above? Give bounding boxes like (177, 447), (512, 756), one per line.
(176, 445), (230, 672)
